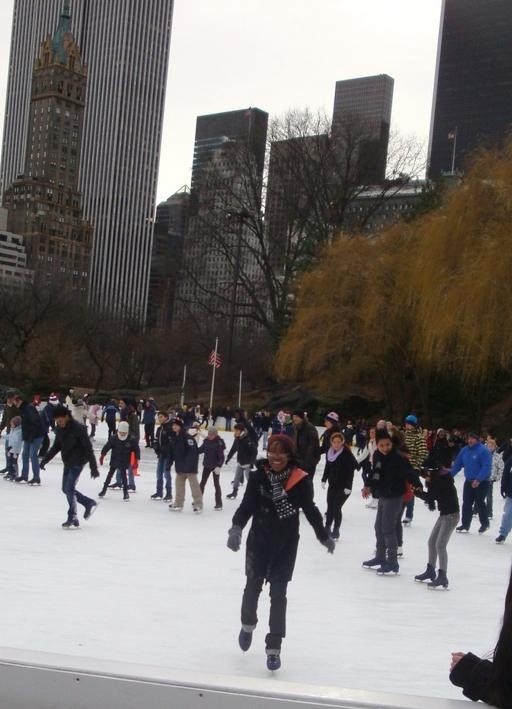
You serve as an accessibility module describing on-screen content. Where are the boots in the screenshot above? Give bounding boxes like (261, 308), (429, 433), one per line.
(426, 568), (449, 588)
(375, 550), (400, 573)
(77, 495), (96, 521)
(413, 562), (436, 580)
(61, 512), (81, 528)
(361, 548), (387, 567)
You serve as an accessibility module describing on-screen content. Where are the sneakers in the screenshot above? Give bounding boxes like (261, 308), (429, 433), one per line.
(266, 654), (283, 670)
(0, 468), (42, 484)
(365, 498), (379, 509)
(323, 524), (340, 539)
(98, 483), (239, 513)
(455, 521), (506, 544)
(236, 628), (253, 653)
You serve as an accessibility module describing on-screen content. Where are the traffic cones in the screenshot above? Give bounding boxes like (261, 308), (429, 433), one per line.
(126, 451), (140, 476)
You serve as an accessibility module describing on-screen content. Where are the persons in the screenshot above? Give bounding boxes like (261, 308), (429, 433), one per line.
(254, 409), (512, 586)
(1, 393), (257, 531)
(449, 566), (512, 709)
(228, 434), (335, 670)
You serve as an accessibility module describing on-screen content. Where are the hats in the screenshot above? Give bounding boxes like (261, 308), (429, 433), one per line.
(403, 414), (418, 426)
(324, 410), (338, 426)
(117, 408), (219, 442)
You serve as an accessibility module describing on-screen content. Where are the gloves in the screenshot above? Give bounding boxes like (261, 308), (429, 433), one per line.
(321, 535), (336, 554)
(343, 488), (351, 496)
(227, 524), (244, 552)
(213, 467), (222, 475)
(320, 482), (327, 490)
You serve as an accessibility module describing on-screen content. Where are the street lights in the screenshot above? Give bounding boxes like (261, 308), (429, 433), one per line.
(223, 209), (252, 391)
